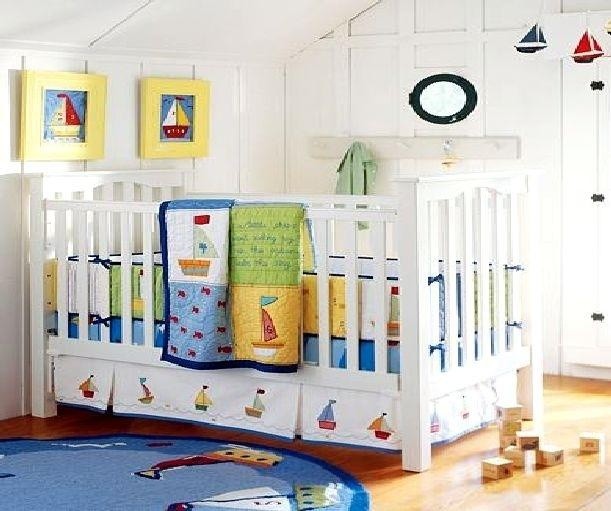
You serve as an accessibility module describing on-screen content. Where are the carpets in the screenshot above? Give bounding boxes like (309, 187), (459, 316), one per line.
(1, 435), (369, 511)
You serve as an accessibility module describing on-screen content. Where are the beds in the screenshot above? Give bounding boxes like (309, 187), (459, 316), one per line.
(30, 169), (545, 471)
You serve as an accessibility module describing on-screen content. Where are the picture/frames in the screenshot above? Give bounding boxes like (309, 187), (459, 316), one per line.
(19, 72), (108, 160)
(140, 77), (210, 159)
